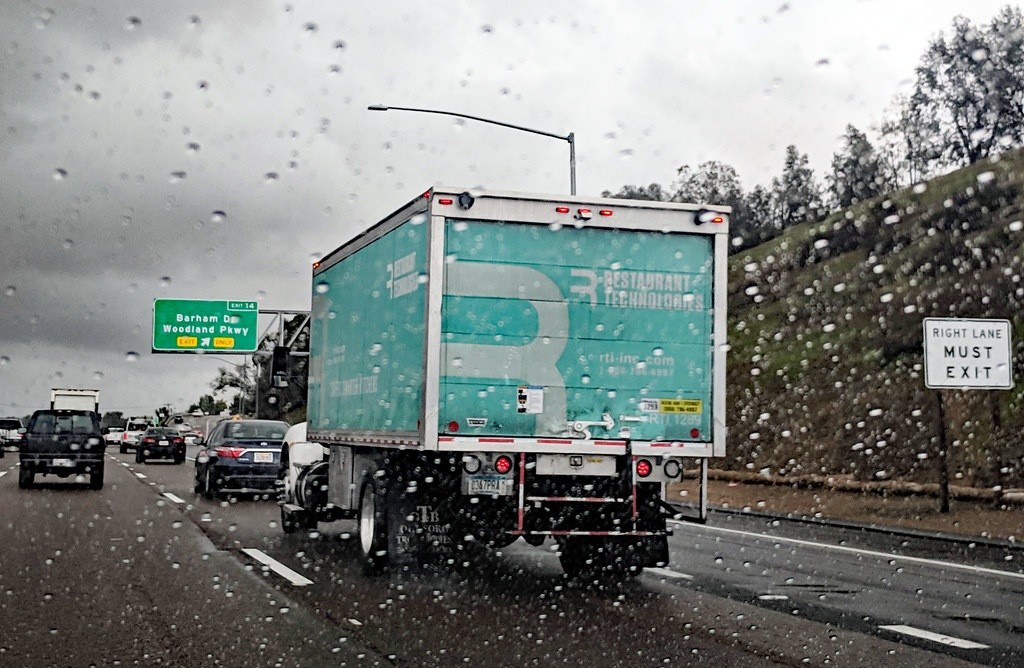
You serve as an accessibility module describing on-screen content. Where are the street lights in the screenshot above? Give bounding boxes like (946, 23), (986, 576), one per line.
(365, 103), (578, 195)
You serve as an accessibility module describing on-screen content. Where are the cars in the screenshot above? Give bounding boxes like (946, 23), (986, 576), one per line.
(193, 420), (292, 502)
(0, 419), (26, 450)
(179, 422), (191, 432)
(105, 427), (124, 446)
(136, 427), (187, 465)
(174, 415), (183, 424)
(183, 433), (199, 446)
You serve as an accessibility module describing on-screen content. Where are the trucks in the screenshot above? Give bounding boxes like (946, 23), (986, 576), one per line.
(277, 186), (734, 596)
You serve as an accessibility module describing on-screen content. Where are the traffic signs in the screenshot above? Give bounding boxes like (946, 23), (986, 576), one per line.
(921, 316), (1013, 390)
(151, 298), (260, 354)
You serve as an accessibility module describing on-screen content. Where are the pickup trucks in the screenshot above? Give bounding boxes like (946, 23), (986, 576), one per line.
(18, 409), (104, 490)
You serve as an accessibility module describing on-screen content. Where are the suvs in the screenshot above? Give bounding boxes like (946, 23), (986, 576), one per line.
(120, 419), (156, 453)
